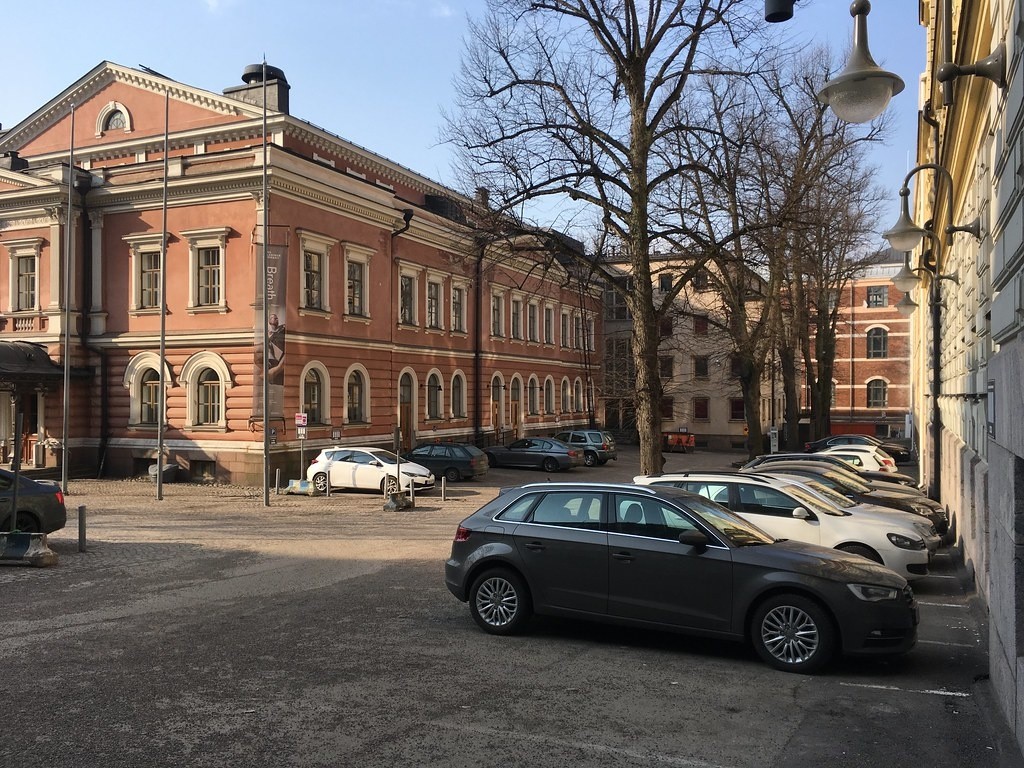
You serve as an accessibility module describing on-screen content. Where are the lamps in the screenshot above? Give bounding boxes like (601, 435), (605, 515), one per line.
(679, 427), (689, 433)
(818, 0), (1008, 125)
(890, 228), (961, 293)
(881, 164), (980, 253)
(715, 360), (722, 367)
(893, 266), (948, 316)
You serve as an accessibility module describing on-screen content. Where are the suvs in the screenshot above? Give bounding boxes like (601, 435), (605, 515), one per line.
(550, 427), (618, 468)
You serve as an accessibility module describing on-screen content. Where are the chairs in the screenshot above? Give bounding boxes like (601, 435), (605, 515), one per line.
(539, 504), (569, 522)
(621, 503), (643, 525)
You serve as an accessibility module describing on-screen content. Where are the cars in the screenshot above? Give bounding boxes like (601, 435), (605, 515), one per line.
(480, 436), (586, 473)
(802, 432), (910, 463)
(698, 442), (950, 556)
(400, 440), (490, 483)
(441, 481), (923, 676)
(306, 447), (436, 498)
(0, 467), (68, 536)
(620, 469), (936, 583)
